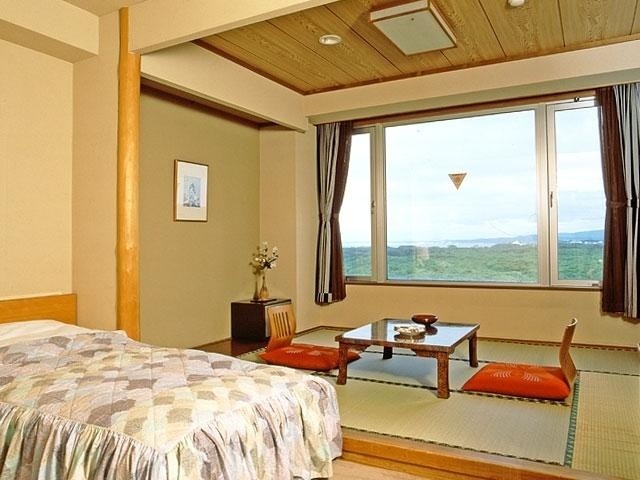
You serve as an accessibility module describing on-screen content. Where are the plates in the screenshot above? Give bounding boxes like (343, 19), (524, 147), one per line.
(393, 326), (429, 336)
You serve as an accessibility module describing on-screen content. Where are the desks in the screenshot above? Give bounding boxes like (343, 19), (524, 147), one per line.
(335, 318), (480, 400)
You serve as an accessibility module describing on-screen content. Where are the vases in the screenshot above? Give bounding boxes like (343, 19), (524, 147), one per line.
(253, 270), (269, 301)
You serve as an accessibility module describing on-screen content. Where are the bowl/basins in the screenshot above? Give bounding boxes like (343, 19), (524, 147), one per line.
(411, 313), (439, 325)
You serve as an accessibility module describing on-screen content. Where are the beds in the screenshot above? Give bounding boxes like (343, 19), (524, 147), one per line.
(1, 292), (340, 479)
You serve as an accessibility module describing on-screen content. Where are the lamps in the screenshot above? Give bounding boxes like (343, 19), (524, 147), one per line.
(369, 0), (458, 57)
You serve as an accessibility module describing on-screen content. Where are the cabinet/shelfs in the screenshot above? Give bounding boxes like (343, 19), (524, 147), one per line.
(231, 298), (291, 343)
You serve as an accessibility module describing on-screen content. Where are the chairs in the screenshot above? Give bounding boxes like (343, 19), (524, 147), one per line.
(260, 303), (361, 375)
(461, 318), (579, 403)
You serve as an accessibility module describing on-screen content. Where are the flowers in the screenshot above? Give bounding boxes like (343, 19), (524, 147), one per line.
(247, 242), (280, 270)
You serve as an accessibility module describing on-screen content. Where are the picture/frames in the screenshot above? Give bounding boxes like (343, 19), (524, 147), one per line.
(174, 159), (209, 222)
